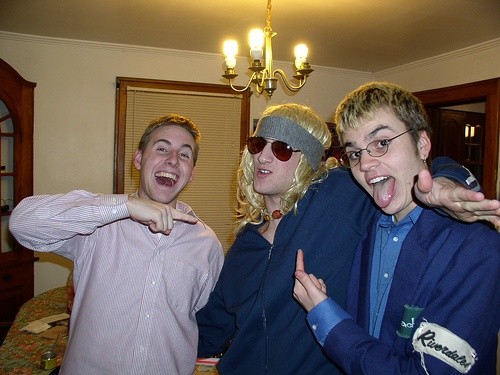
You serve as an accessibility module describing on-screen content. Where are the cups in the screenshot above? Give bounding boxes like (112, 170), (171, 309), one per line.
(41, 351), (57, 370)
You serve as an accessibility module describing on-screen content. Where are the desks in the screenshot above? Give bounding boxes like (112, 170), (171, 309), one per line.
(0, 285), (219, 375)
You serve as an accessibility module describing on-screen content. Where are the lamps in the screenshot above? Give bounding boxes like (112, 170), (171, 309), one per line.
(221, 0), (315, 98)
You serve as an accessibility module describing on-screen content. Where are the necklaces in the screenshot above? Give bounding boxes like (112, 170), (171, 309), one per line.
(263, 208), (283, 221)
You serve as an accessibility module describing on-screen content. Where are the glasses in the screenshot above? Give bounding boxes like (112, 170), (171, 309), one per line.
(338, 128), (412, 168)
(245, 136), (301, 162)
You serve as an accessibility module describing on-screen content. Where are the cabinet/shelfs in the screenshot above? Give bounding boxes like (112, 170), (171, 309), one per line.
(0, 58), (36, 345)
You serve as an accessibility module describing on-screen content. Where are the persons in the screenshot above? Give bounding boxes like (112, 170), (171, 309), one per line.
(9, 114), (225, 375)
(67, 103), (500, 375)
(293, 81), (500, 375)
